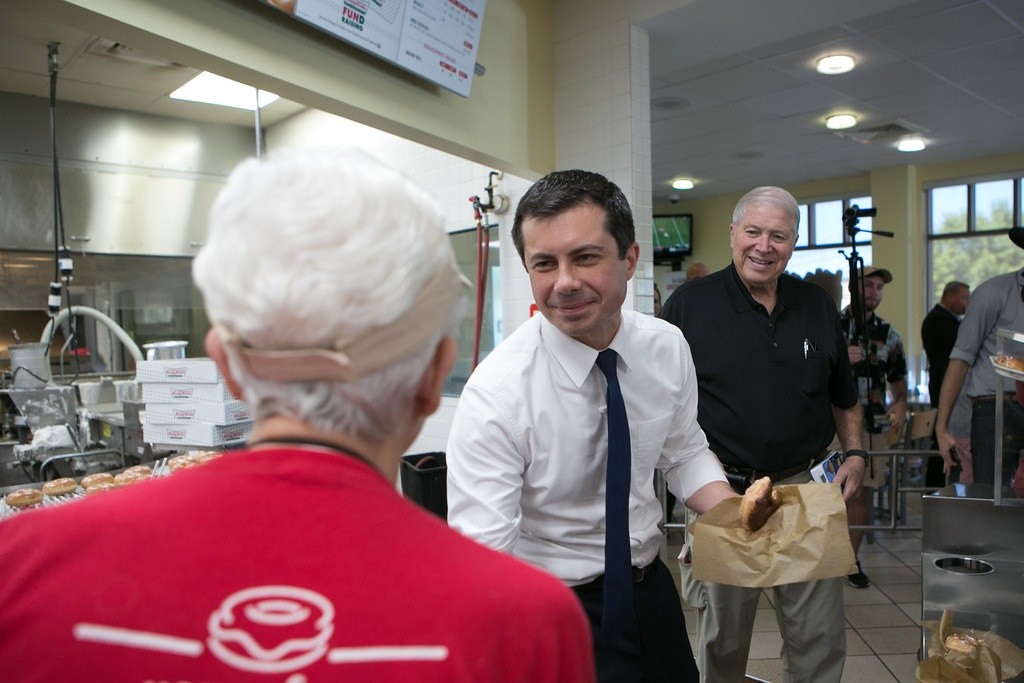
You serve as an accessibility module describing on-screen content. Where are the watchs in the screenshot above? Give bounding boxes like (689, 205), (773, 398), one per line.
(845, 450), (869, 467)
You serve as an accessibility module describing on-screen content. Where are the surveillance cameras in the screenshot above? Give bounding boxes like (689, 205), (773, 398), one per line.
(670, 195), (680, 204)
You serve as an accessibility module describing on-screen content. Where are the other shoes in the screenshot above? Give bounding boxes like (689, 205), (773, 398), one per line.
(844, 562), (871, 588)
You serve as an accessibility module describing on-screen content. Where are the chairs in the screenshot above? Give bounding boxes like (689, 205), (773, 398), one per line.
(872, 408), (939, 525)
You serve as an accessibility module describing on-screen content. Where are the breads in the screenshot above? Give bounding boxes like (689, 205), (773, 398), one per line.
(993, 354), (1024, 373)
(5, 448), (223, 509)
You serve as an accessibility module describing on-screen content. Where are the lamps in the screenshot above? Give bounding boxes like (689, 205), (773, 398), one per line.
(816, 56), (856, 75)
(826, 115), (858, 129)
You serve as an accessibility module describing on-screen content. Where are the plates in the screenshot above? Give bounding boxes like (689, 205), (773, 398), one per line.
(990, 356), (1024, 380)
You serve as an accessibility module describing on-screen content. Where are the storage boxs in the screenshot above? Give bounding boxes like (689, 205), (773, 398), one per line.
(136, 357), (227, 383)
(143, 421), (254, 447)
(142, 381), (235, 403)
(138, 399), (257, 426)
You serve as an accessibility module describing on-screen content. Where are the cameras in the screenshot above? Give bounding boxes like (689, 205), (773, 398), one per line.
(845, 205), (876, 220)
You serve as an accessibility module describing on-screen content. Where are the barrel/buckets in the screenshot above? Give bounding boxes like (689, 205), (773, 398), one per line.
(8, 341), (54, 389)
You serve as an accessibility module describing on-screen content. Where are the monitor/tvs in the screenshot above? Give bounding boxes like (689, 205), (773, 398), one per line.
(652, 213), (693, 255)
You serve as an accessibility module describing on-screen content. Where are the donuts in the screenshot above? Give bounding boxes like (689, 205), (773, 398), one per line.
(738, 476), (783, 533)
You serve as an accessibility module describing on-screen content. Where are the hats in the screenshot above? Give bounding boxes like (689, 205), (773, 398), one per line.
(220, 253), (471, 377)
(858, 265), (894, 286)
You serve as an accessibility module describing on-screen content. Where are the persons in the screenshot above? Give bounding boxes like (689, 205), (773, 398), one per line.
(921, 226), (1024, 495)
(657, 187), (868, 683)
(0, 138), (597, 683)
(653, 284), (662, 317)
(686, 262), (708, 283)
(446, 169), (743, 683)
(828, 267), (907, 589)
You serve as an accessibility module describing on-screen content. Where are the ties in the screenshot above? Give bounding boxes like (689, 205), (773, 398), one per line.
(591, 348), (633, 595)
